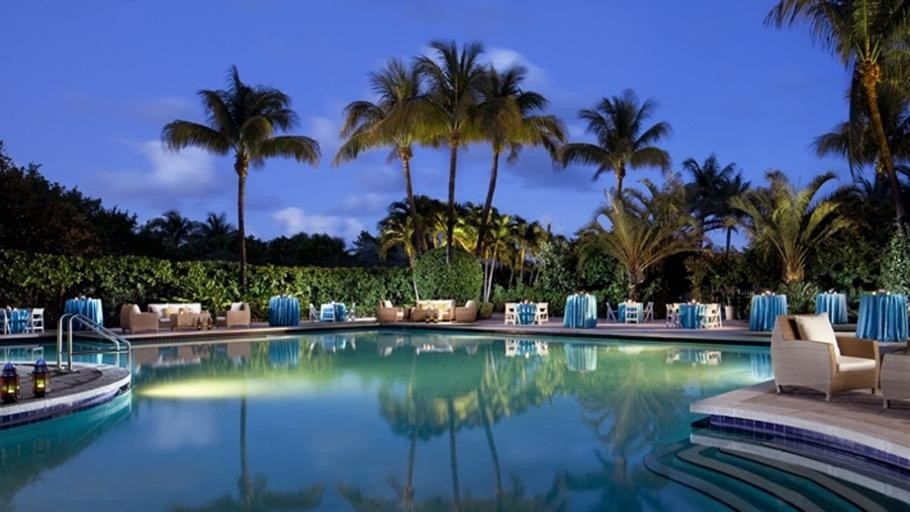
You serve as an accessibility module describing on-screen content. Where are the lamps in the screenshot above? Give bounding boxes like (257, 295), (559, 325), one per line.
(0, 362), (21, 403)
(31, 359), (52, 398)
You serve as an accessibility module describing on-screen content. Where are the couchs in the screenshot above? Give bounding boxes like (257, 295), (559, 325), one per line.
(148, 303), (202, 328)
(410, 299), (455, 321)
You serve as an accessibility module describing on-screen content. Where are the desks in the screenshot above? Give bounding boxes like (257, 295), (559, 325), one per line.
(750, 352), (772, 379)
(65, 299), (104, 331)
(170, 313), (212, 330)
(854, 294), (909, 342)
(564, 345), (597, 372)
(815, 294), (848, 323)
(562, 295), (598, 328)
(268, 295), (300, 326)
(516, 304), (537, 325)
(750, 295), (787, 331)
(268, 337), (299, 368)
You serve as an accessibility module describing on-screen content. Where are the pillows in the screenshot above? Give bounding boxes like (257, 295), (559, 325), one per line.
(384, 301), (392, 308)
(230, 302), (243, 312)
(795, 313), (842, 357)
(128, 303), (142, 314)
(466, 301), (473, 308)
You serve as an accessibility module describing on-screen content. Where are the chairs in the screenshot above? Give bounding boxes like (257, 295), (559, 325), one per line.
(505, 337), (517, 359)
(121, 303), (160, 334)
(665, 350), (724, 366)
(531, 302), (549, 326)
(504, 303), (521, 326)
(535, 341), (549, 358)
(455, 299), (477, 323)
(880, 339), (910, 408)
(308, 303), (357, 323)
(606, 302), (723, 329)
(375, 299), (404, 322)
(770, 314), (881, 402)
(226, 302), (252, 329)
(0, 306), (45, 336)
